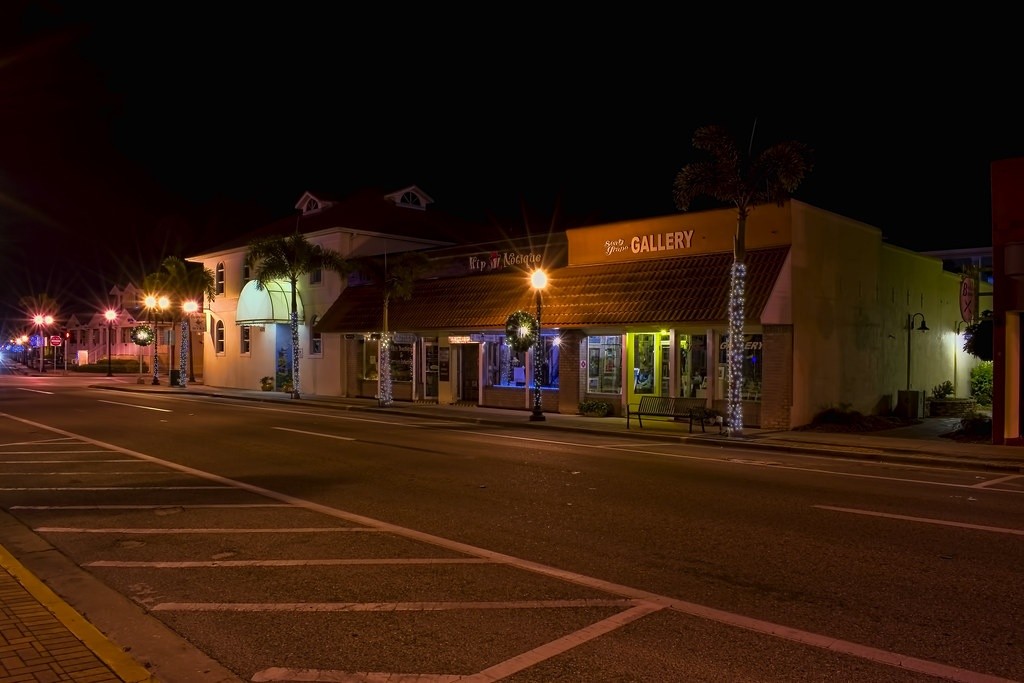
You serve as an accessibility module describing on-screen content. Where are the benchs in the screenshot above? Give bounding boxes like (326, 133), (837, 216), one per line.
(627, 396), (708, 434)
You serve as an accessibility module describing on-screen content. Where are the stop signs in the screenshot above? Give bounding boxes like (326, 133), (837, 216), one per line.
(50, 335), (62, 346)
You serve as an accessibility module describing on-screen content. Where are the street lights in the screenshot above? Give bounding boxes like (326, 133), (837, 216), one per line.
(529, 267), (549, 421)
(15, 334), (29, 366)
(145, 295), (160, 385)
(158, 296), (170, 375)
(103, 309), (117, 377)
(182, 300), (198, 382)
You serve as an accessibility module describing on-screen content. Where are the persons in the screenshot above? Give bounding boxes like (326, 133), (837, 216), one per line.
(641, 360), (655, 387)
(680, 348), (687, 372)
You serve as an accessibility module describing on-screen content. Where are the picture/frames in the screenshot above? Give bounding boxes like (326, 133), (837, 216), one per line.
(719, 366), (725, 379)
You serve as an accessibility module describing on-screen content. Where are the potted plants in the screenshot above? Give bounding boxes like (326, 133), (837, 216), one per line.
(576, 399), (610, 418)
(260, 376), (274, 391)
(281, 379), (293, 392)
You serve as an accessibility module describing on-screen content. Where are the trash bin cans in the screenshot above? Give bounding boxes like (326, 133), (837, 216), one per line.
(170, 369), (180, 386)
(898, 390), (918, 421)
